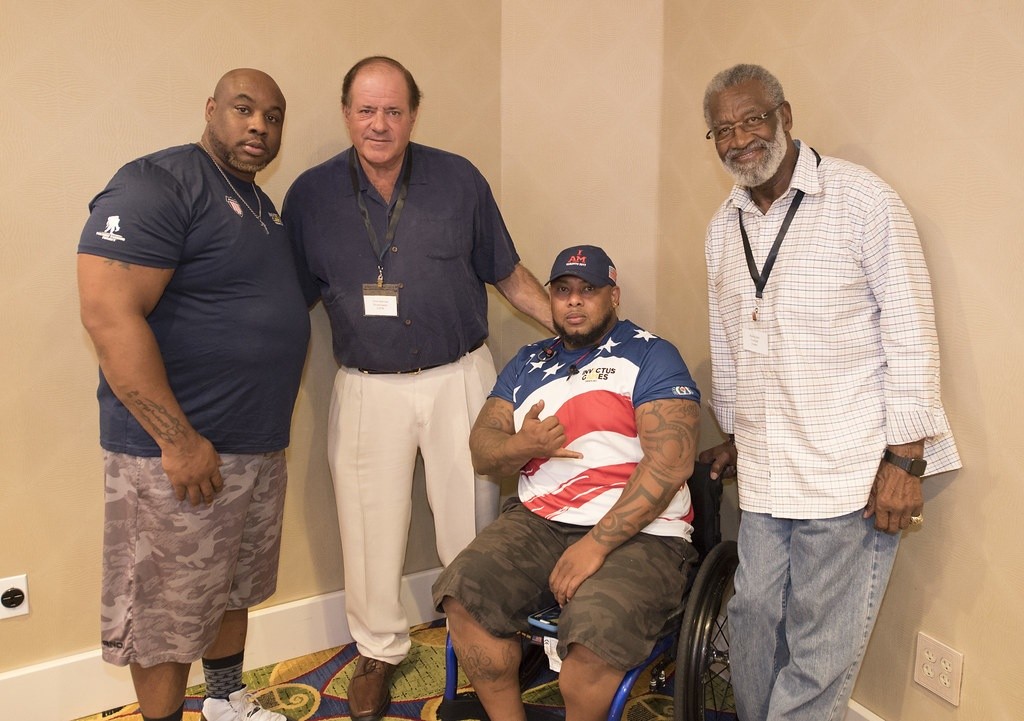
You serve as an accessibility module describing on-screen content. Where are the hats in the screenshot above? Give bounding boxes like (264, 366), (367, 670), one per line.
(545, 246), (619, 289)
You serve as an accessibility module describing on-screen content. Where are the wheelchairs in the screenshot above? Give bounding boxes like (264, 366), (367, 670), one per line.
(436, 462), (739, 721)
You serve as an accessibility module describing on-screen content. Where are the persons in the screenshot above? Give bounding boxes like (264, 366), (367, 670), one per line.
(697, 64), (964, 721)
(281, 56), (560, 721)
(432, 244), (699, 721)
(76, 69), (311, 721)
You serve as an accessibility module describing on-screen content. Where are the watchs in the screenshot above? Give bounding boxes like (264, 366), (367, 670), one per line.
(884, 450), (928, 476)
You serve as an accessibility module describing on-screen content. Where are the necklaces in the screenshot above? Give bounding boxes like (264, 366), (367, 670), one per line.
(199, 141), (270, 235)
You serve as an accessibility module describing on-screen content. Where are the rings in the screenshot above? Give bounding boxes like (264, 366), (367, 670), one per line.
(910, 512), (924, 526)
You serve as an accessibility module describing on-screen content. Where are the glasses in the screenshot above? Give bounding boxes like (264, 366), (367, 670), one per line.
(705, 101), (786, 141)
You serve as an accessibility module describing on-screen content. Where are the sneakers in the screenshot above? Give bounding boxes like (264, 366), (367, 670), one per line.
(201, 684), (293, 720)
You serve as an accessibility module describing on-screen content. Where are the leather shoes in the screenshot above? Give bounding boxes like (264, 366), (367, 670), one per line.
(347, 654), (402, 721)
